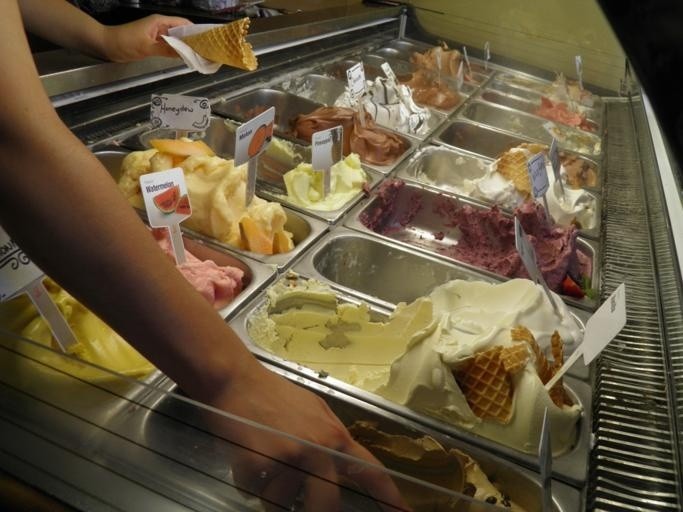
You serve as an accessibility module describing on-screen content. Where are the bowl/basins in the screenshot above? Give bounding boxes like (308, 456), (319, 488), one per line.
(390, 38), (495, 79)
(437, 112), (599, 198)
(344, 176), (600, 311)
(487, 74), (601, 129)
(80, 149), (332, 272)
(211, 86), (419, 175)
(393, 138), (599, 240)
(349, 52), (481, 98)
(479, 86), (602, 141)
(282, 68), (448, 142)
(287, 220), (598, 386)
(456, 94), (600, 161)
(1, 268), (177, 407)
(314, 59), (469, 115)
(139, 115), (385, 226)
(139, 339), (580, 512)
(119, 205), (278, 323)
(209, 269), (590, 490)
(373, 45), (489, 89)
(492, 69), (603, 113)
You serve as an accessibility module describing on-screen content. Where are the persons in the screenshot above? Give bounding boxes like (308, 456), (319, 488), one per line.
(0, 0), (410, 511)
